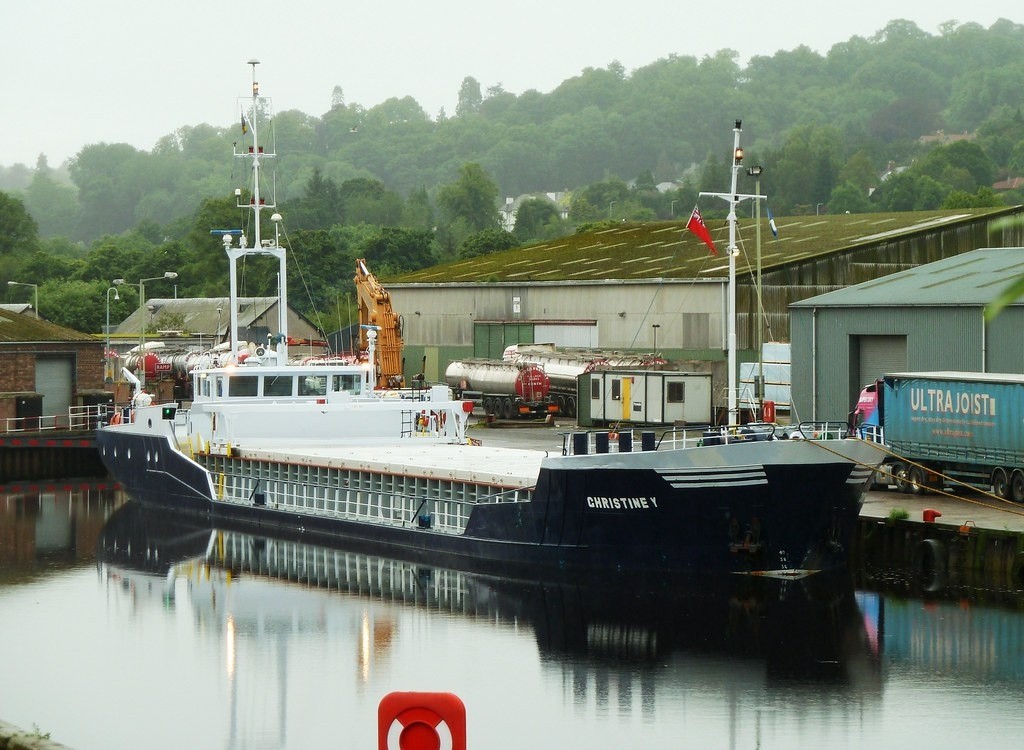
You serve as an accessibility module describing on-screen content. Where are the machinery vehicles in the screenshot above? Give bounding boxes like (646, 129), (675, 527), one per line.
(353, 258), (406, 388)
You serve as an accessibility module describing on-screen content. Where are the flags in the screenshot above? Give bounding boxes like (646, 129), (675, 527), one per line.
(767, 208), (778, 236)
(687, 205), (718, 255)
(241, 116), (247, 134)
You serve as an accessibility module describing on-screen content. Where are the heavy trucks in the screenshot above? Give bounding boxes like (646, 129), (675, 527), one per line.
(502, 342), (668, 417)
(288, 355), (349, 394)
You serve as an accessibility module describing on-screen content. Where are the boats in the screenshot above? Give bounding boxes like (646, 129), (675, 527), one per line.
(97, 499), (895, 750)
(96, 61), (893, 593)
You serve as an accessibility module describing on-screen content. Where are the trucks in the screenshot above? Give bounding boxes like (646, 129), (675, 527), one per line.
(847, 372), (1024, 502)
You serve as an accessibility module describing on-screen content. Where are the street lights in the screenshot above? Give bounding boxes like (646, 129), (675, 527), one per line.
(610, 200), (618, 220)
(138, 271), (178, 382)
(107, 287), (120, 362)
(267, 333), (272, 366)
(671, 200), (678, 215)
(112, 279), (146, 385)
(652, 323), (660, 371)
(8, 281), (38, 318)
(817, 202), (824, 216)
